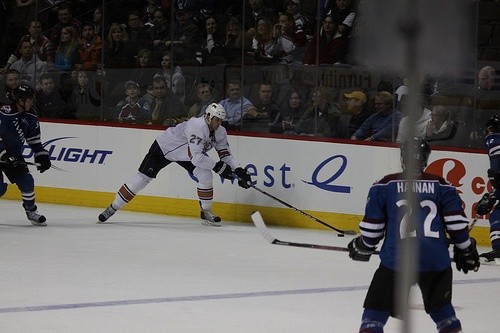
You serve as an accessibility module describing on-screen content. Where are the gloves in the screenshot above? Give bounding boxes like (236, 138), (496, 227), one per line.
(452, 238), (480, 273)
(235, 167), (253, 189)
(35, 150), (51, 173)
(475, 193), (496, 217)
(347, 236), (376, 262)
(6, 155), (25, 171)
(212, 161), (233, 179)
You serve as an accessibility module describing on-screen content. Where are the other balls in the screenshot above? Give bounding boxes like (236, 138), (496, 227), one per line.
(337, 234), (344, 237)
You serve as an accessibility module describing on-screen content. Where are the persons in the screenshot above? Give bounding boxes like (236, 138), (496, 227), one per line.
(97, 103), (251, 224)
(348, 137), (481, 333)
(475, 116), (500, 264)
(0, 84), (51, 224)
(0, 0), (500, 146)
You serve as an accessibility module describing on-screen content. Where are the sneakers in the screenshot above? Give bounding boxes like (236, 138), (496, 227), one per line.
(23, 205), (47, 226)
(479, 250), (500, 266)
(97, 204), (117, 224)
(200, 210), (223, 226)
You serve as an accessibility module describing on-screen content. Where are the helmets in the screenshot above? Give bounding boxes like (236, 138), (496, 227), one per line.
(400, 137), (431, 168)
(13, 85), (36, 104)
(206, 102), (227, 122)
(486, 114), (500, 136)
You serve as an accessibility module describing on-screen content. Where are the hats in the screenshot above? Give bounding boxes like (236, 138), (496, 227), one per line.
(124, 79), (141, 89)
(343, 91), (367, 102)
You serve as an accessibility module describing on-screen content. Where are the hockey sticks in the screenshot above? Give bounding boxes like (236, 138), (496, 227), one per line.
(448, 214), (480, 245)
(231, 173), (356, 235)
(250, 210), (475, 265)
(16, 161), (64, 173)
